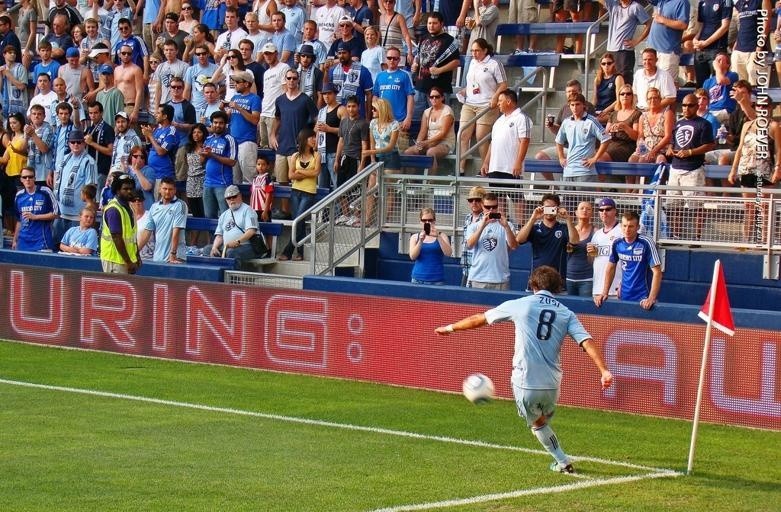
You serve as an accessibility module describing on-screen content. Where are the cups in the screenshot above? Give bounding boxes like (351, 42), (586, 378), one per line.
(566, 242), (573, 253)
(327, 55), (335, 67)
(185, 32), (195, 45)
(466, 16), (476, 30)
(587, 242), (599, 257)
(547, 115), (555, 127)
(156, 36), (166, 49)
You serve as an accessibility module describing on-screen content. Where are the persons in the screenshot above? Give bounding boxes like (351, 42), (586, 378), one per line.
(0, 0), (781, 311)
(434, 264), (613, 476)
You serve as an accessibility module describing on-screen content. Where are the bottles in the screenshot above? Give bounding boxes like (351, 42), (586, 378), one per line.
(718, 124), (727, 144)
(219, 84), (226, 101)
(638, 138), (647, 157)
(774, 40), (781, 60)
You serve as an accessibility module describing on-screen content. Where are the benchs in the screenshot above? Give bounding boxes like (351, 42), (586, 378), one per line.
(504, 159), (741, 216)
(400, 119), (473, 178)
(185, 217), (283, 273)
(257, 147), (434, 212)
(406, 51), (561, 108)
(175, 179), (330, 245)
(415, 20), (599, 76)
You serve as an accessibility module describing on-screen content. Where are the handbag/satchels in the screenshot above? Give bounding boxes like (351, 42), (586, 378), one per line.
(249, 233), (269, 258)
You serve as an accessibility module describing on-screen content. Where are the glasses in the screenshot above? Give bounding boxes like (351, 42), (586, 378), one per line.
(194, 52), (244, 84)
(387, 56), (400, 61)
(597, 207), (613, 212)
(467, 198), (498, 210)
(170, 85), (183, 90)
(592, 60), (771, 109)
(21, 175), (36, 179)
(132, 154), (147, 160)
(286, 76), (299, 81)
(428, 94), (442, 99)
(181, 7), (192, 11)
(420, 218), (436, 222)
(8, 112), (17, 117)
(227, 195), (239, 200)
(71, 142), (82, 144)
(118, 175), (134, 183)
(118, 26), (158, 65)
(131, 198), (144, 203)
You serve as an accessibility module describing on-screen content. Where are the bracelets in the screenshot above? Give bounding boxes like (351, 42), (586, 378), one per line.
(445, 324), (455, 334)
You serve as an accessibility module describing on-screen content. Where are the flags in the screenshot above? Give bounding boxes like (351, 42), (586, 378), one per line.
(698, 258), (736, 337)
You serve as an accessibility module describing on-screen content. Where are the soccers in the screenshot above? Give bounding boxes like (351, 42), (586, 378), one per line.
(463, 373), (493, 404)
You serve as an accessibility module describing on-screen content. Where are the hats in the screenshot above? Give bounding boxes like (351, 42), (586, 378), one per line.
(65, 47), (112, 74)
(297, 15), (353, 94)
(262, 43), (278, 54)
(598, 198), (615, 207)
(165, 12), (179, 22)
(229, 72), (253, 85)
(467, 186), (486, 200)
(114, 110), (129, 121)
(68, 130), (85, 143)
(224, 185), (239, 197)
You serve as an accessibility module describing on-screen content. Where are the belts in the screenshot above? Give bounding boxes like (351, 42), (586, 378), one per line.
(123, 103), (136, 107)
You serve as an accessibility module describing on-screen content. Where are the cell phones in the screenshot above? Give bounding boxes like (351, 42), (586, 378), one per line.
(489, 212), (501, 219)
(223, 102), (229, 108)
(543, 207), (558, 215)
(424, 222), (430, 235)
(318, 120), (323, 124)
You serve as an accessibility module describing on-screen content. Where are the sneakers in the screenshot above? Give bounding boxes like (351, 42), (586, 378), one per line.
(513, 47), (536, 55)
(272, 210), (292, 219)
(278, 255), (304, 260)
(550, 461), (575, 475)
(335, 214), (370, 228)
